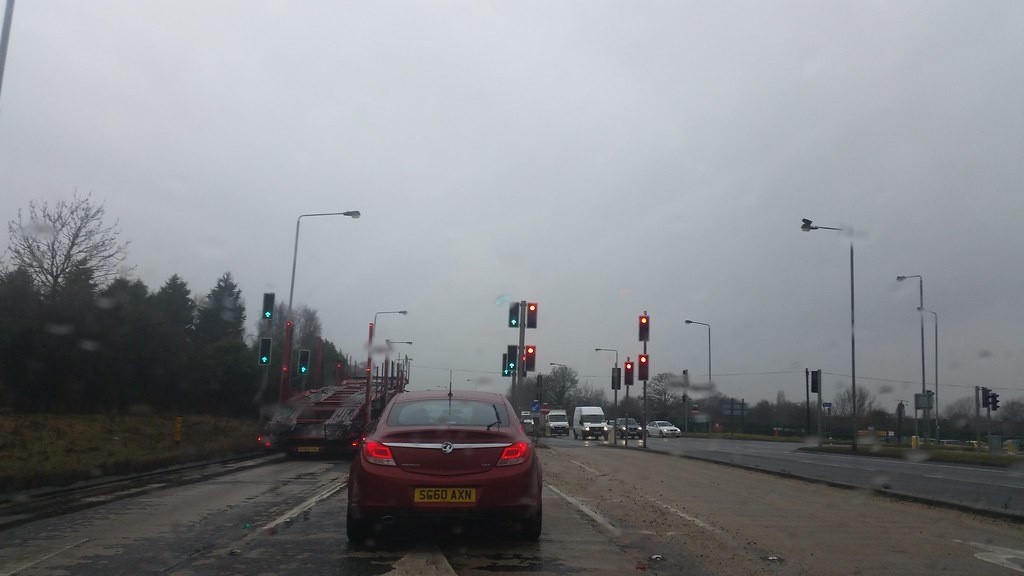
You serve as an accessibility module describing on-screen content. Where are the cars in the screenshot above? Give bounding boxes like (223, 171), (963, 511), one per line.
(920, 437), (1024, 454)
(345, 388), (542, 541)
(521, 406), (681, 442)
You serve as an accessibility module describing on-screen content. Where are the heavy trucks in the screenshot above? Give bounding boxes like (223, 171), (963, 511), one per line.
(256, 351), (411, 461)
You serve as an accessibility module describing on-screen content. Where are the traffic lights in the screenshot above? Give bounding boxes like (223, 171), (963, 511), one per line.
(527, 303), (537, 329)
(507, 345), (517, 370)
(258, 337), (271, 365)
(624, 362), (633, 385)
(262, 293), (274, 319)
(502, 353), (511, 377)
(639, 315), (650, 341)
(982, 387), (992, 408)
(991, 393), (1000, 411)
(299, 350), (309, 375)
(525, 345), (536, 372)
(639, 354), (648, 381)
(509, 302), (519, 327)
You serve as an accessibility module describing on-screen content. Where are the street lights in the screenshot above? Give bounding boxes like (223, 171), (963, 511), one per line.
(799, 216), (941, 448)
(365, 310), (413, 424)
(685, 319), (714, 437)
(281, 210), (361, 406)
(594, 348), (618, 445)
(550, 362), (566, 410)
